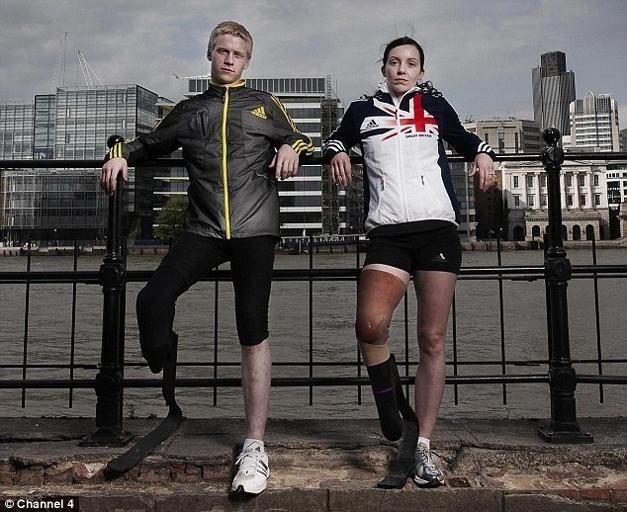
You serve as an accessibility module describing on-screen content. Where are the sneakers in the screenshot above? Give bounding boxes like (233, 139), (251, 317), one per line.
(411, 442), (445, 488)
(230, 450), (271, 495)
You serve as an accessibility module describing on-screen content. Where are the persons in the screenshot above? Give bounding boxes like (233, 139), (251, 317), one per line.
(320, 37), (496, 488)
(100, 20), (314, 498)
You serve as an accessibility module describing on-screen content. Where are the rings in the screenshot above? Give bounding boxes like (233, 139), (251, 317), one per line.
(489, 172), (493, 175)
